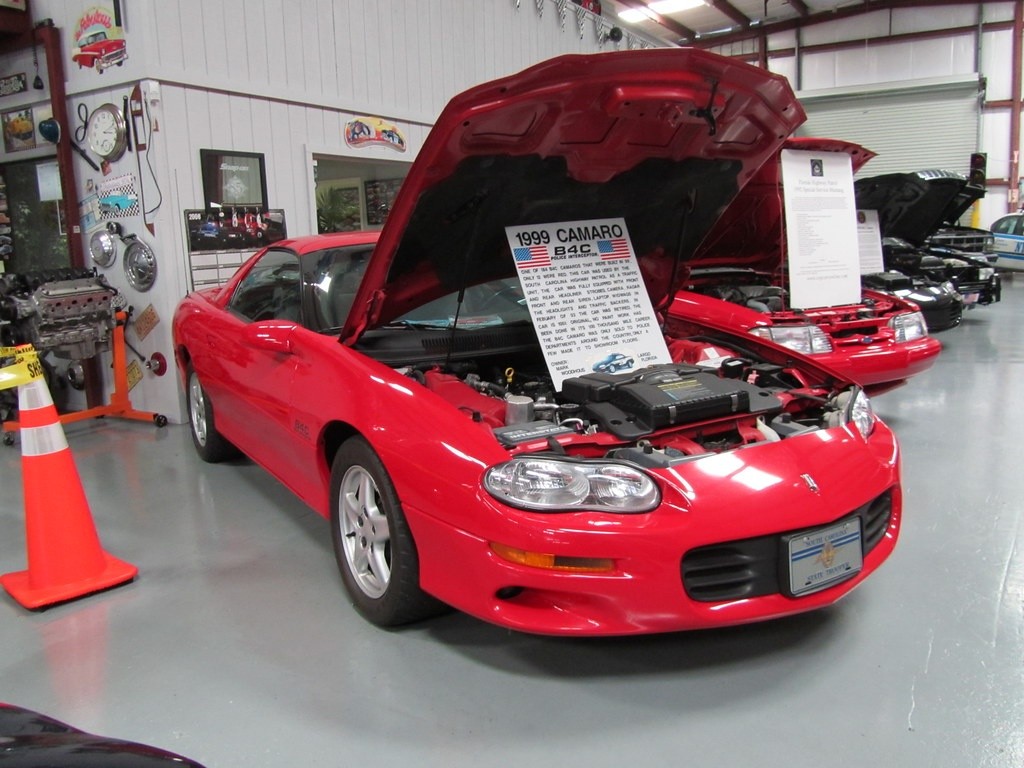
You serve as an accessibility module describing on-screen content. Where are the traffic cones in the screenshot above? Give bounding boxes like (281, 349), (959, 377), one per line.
(0, 342), (141, 613)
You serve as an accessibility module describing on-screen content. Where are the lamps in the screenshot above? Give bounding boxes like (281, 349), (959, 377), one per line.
(87, 102), (129, 163)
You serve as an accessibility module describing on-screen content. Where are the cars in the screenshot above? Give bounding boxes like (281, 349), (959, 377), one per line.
(171, 46), (1024, 636)
